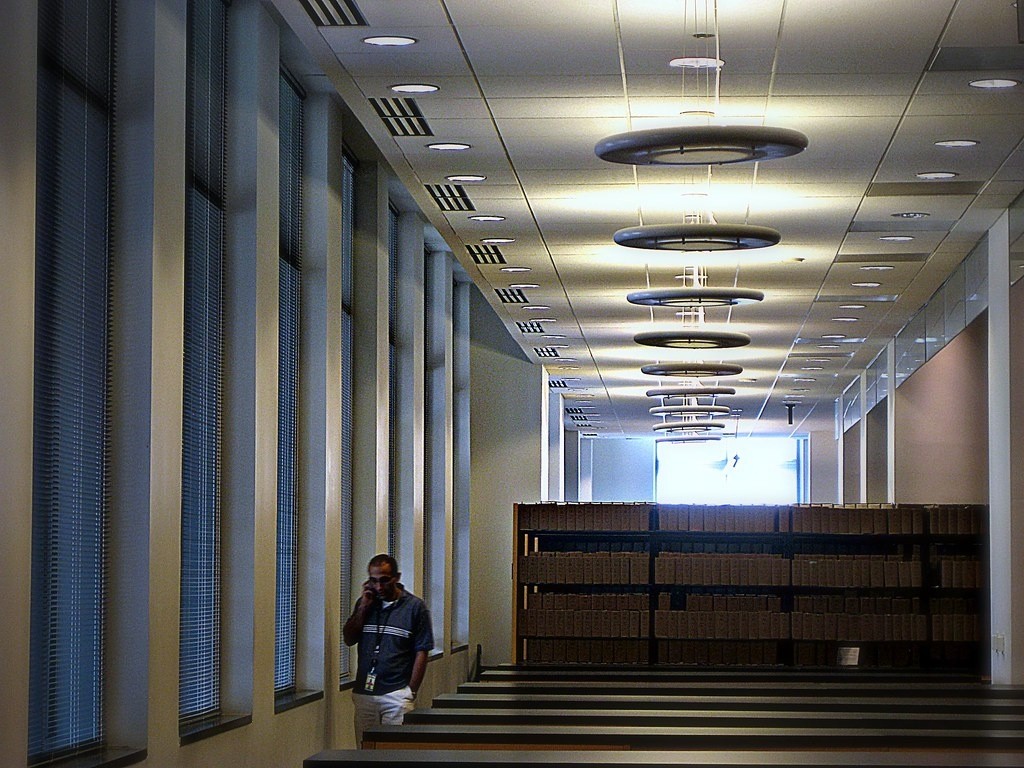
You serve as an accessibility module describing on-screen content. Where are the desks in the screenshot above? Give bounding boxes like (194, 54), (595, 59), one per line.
(302, 669), (1024, 768)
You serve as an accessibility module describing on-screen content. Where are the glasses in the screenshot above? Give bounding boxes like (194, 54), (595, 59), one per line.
(369, 573), (398, 584)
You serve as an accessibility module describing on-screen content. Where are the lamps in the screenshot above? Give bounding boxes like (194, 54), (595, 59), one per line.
(590, 122), (808, 166)
(652, 422), (727, 433)
(639, 362), (742, 378)
(645, 386), (737, 400)
(656, 434), (723, 445)
(649, 404), (733, 418)
(632, 331), (750, 350)
(361, 35), (419, 46)
(626, 286), (767, 309)
(611, 222), (781, 253)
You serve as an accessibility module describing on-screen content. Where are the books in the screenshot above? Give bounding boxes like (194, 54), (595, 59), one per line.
(508, 503), (990, 645)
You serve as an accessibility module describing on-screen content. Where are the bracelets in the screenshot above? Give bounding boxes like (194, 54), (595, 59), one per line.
(412, 690), (418, 696)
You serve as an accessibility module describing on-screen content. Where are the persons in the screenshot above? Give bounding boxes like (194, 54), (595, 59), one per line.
(340, 553), (434, 754)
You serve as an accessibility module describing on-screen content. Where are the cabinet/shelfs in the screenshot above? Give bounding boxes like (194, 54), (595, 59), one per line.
(509, 500), (989, 681)
